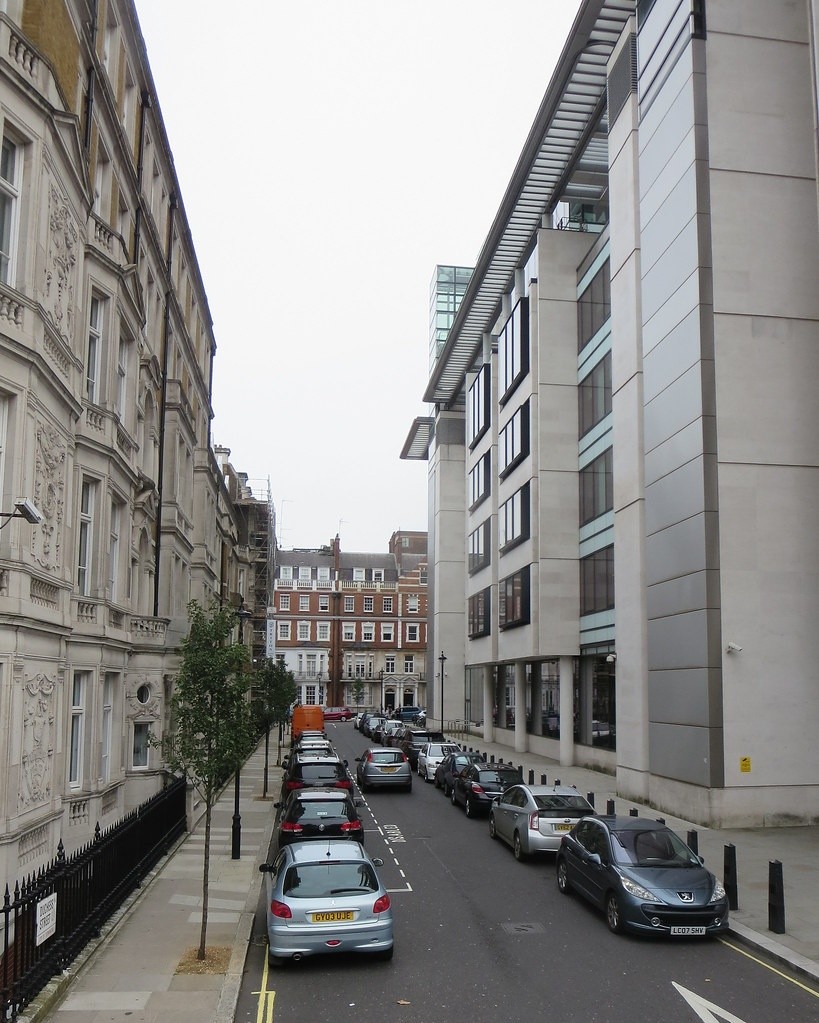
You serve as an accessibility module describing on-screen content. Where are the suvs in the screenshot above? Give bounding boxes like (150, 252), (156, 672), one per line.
(280, 751), (354, 803)
(322, 707), (352, 722)
(387, 705), (421, 723)
(401, 730), (446, 772)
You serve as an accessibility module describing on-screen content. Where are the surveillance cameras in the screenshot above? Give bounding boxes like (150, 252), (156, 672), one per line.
(606, 656), (614, 665)
(13, 497), (45, 524)
(728, 641), (742, 651)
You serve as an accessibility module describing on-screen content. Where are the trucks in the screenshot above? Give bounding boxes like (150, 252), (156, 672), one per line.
(289, 704), (326, 738)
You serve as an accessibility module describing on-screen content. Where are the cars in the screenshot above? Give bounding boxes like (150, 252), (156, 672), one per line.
(288, 730), (337, 753)
(258, 839), (394, 964)
(416, 742), (466, 783)
(355, 746), (413, 792)
(487, 784), (603, 861)
(354, 711), (409, 748)
(433, 752), (489, 796)
(449, 762), (527, 817)
(554, 816), (732, 938)
(272, 787), (366, 849)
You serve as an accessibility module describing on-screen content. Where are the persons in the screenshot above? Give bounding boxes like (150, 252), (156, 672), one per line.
(382, 706), (401, 720)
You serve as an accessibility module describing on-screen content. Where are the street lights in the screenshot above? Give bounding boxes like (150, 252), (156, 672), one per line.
(379, 668), (385, 715)
(437, 650), (448, 737)
(317, 670), (323, 707)
(230, 597), (253, 860)
